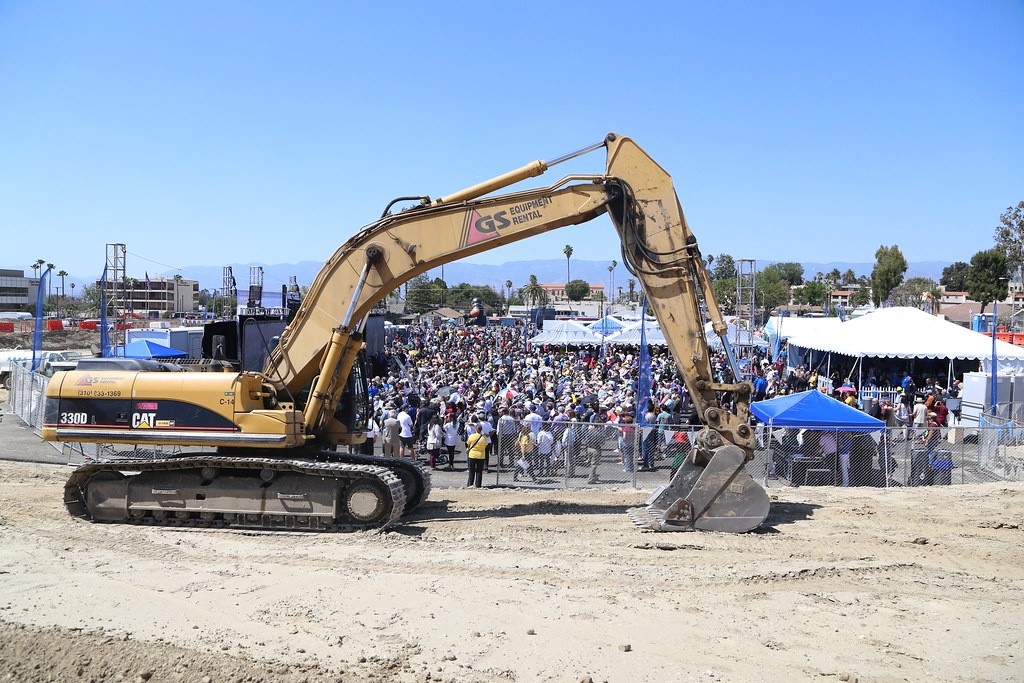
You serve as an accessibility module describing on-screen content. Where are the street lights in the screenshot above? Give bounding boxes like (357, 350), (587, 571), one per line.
(1000, 277), (1016, 327)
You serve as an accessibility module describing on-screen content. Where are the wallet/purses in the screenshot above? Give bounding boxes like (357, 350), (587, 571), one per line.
(517, 457), (530, 470)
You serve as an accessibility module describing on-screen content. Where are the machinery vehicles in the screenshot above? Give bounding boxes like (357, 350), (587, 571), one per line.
(42, 132), (771, 533)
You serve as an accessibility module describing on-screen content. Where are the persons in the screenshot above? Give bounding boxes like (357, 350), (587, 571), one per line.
(353, 318), (691, 489)
(711, 346), (968, 450)
(782, 427), (851, 490)
(978, 361), (984, 371)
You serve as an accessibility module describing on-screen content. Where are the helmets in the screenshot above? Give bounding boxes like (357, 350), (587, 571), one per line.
(900, 392), (907, 396)
(897, 386), (902, 391)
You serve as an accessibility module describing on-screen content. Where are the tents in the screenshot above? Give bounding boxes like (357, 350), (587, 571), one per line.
(526, 306), (1024, 402)
(749, 388), (891, 488)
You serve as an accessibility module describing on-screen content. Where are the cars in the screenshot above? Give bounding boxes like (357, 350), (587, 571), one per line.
(1, 351), (193, 390)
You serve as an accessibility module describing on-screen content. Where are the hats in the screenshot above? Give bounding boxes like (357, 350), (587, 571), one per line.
(543, 353), (548, 356)
(532, 398), (542, 405)
(930, 412), (937, 417)
(529, 405), (536, 410)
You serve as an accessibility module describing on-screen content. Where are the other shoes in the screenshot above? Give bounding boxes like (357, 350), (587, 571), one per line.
(466, 484), (482, 490)
(587, 473), (599, 484)
(531, 478), (539, 484)
(430, 466), (436, 470)
(443, 466), (454, 471)
(638, 465), (655, 472)
(513, 477), (520, 482)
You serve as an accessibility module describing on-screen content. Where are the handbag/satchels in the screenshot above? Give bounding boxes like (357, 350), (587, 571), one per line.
(466, 448), (469, 454)
